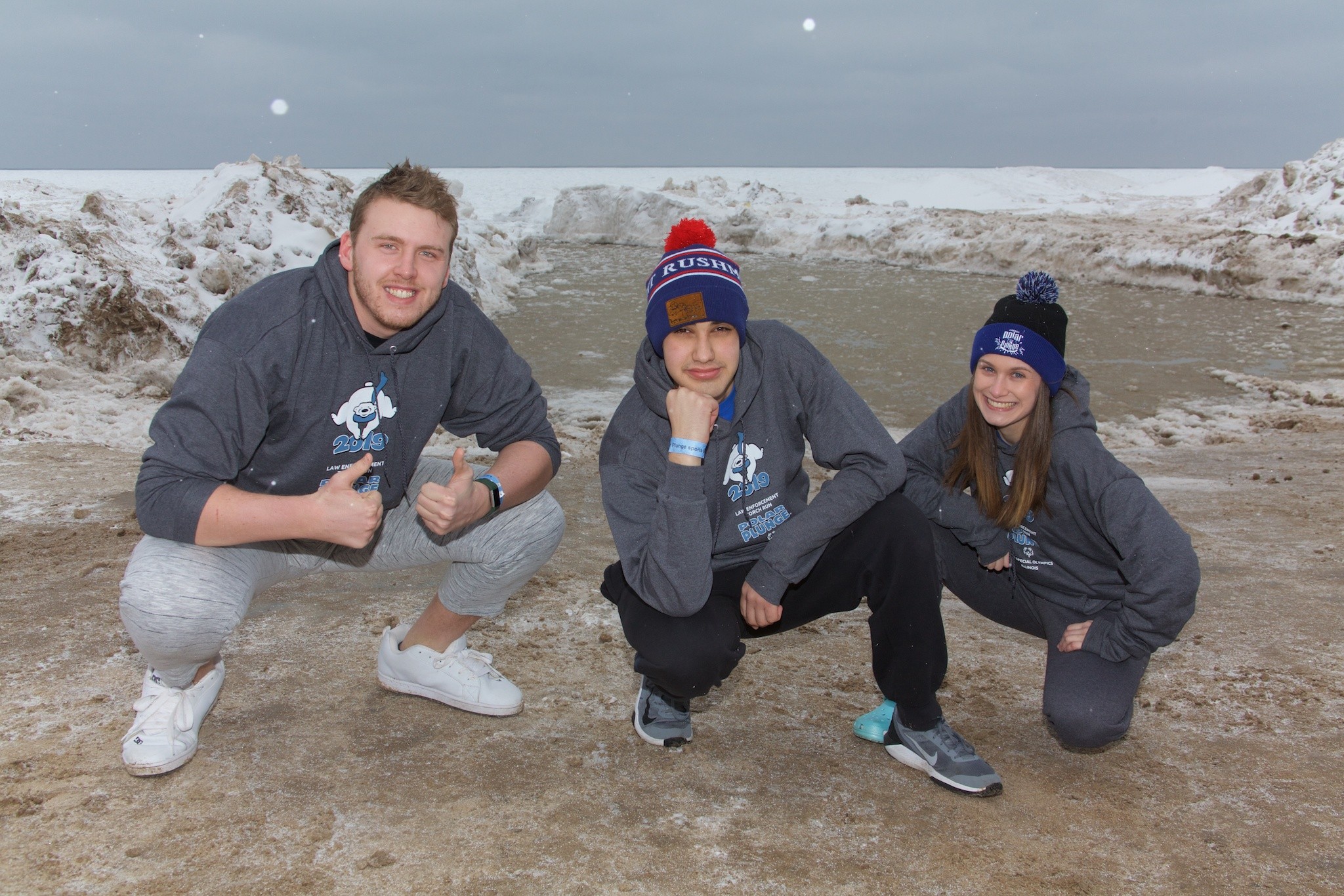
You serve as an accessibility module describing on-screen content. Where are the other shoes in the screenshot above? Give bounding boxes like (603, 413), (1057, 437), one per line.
(853, 698), (897, 743)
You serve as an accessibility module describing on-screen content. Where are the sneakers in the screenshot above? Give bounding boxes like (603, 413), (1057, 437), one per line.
(376, 624), (525, 717)
(121, 653), (225, 777)
(632, 674), (694, 748)
(883, 704), (1004, 798)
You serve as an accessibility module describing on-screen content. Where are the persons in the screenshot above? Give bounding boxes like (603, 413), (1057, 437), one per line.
(119, 159), (563, 776)
(851, 270), (1202, 747)
(598, 215), (1006, 796)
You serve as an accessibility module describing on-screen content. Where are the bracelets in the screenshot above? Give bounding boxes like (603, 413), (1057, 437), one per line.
(478, 475), (505, 504)
(668, 436), (707, 458)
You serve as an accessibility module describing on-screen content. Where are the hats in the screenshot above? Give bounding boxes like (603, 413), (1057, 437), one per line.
(645, 217), (749, 360)
(970, 269), (1068, 400)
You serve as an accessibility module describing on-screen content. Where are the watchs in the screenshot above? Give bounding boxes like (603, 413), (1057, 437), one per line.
(475, 479), (501, 519)
